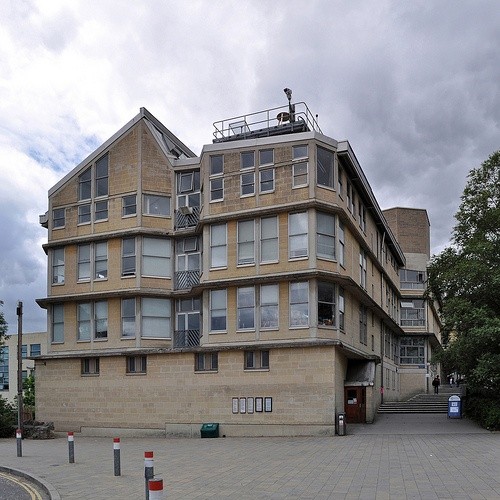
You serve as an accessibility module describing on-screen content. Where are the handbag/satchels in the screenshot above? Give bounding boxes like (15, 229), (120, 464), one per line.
(432, 381), (439, 386)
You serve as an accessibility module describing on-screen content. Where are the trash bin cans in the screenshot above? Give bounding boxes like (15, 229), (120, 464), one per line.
(336, 412), (346, 436)
(200, 422), (219, 438)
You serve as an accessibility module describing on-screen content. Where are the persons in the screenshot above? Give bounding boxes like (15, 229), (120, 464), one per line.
(319, 318), (332, 325)
(433, 375), (440, 394)
(447, 374), (465, 384)
